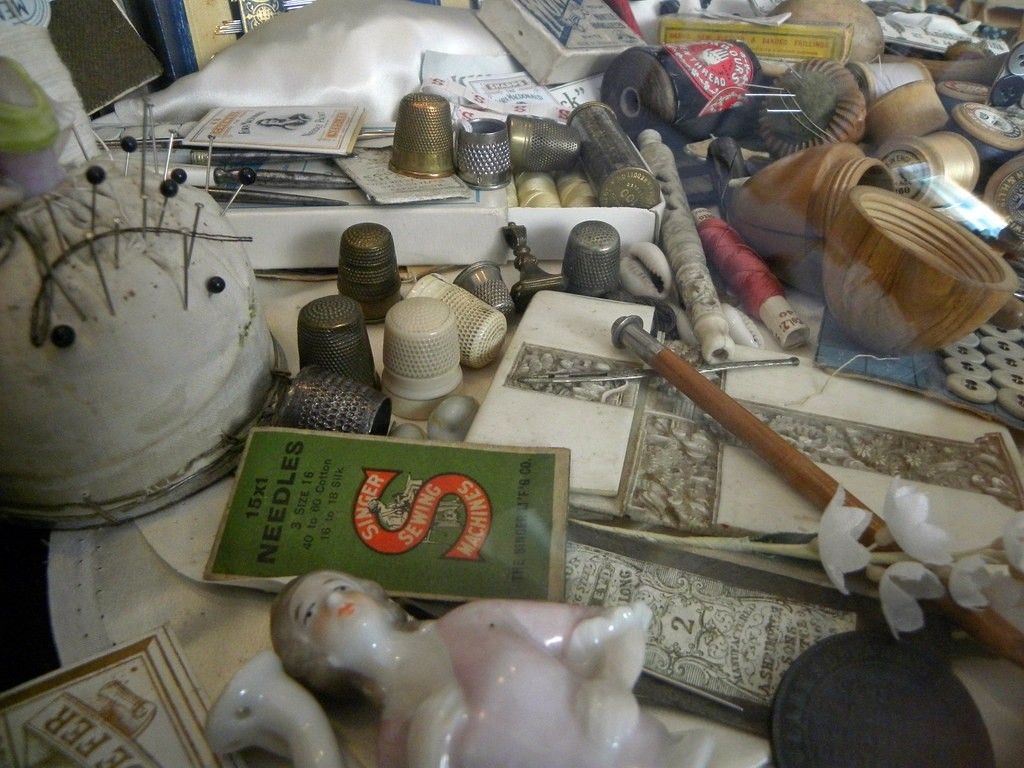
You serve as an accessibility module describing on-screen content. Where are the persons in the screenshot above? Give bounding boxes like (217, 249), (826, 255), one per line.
(204, 570), (765, 767)
